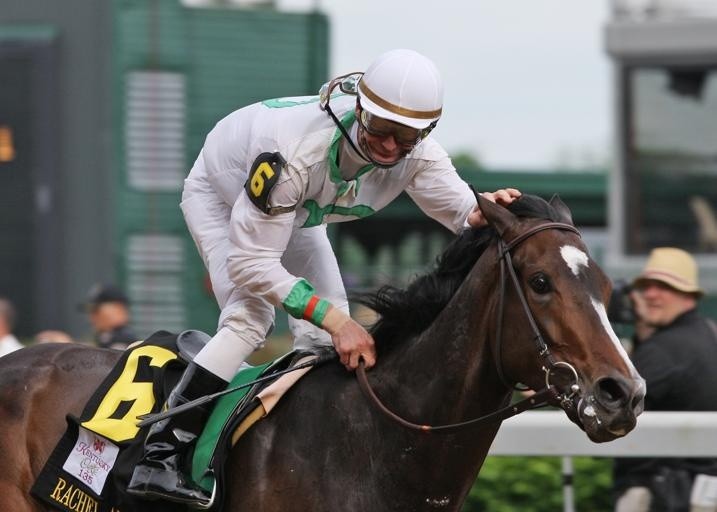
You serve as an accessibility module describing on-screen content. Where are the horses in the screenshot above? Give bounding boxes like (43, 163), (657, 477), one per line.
(0, 182), (647, 512)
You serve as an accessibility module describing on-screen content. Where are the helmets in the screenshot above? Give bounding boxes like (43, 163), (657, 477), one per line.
(357, 48), (442, 129)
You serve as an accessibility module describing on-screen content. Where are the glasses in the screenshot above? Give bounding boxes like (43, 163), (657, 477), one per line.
(319, 72), (372, 162)
(360, 109), (437, 146)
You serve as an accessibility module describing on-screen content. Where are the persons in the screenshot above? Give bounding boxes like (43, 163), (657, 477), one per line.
(127, 48), (521, 504)
(0, 296), (24, 357)
(76, 285), (133, 351)
(612, 247), (717, 511)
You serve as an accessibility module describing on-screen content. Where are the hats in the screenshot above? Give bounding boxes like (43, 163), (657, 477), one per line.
(90, 282), (129, 304)
(635, 247), (703, 297)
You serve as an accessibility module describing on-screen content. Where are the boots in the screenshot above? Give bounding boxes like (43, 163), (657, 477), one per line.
(127, 360), (230, 505)
